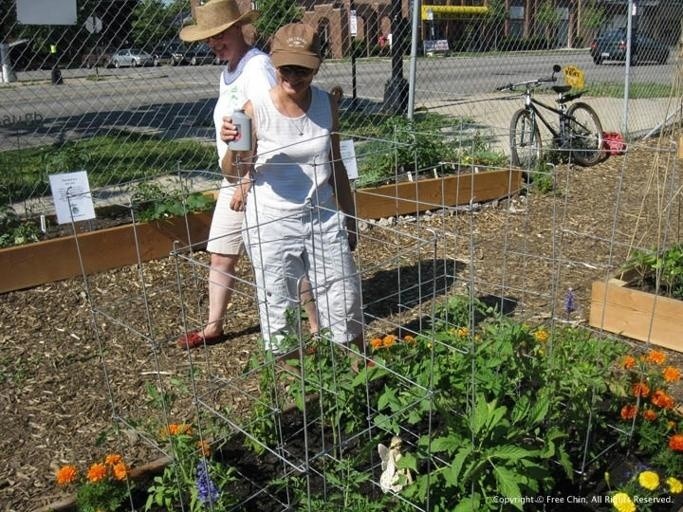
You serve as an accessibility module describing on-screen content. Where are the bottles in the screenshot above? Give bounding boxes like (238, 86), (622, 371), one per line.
(229, 109), (251, 151)
(564, 285), (576, 314)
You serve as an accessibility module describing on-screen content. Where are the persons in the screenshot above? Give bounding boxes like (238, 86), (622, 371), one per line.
(179, 1), (318, 352)
(219, 20), (368, 405)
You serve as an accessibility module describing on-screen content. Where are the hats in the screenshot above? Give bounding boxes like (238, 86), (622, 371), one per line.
(178, 0), (261, 43)
(270, 23), (322, 70)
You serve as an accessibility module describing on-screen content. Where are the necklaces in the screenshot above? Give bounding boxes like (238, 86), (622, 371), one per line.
(277, 86), (311, 136)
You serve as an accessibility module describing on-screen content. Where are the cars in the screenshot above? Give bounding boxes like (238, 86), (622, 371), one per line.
(82, 44), (216, 69)
(590, 29), (670, 65)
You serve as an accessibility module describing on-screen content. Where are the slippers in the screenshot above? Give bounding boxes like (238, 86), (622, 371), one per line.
(178, 332), (224, 349)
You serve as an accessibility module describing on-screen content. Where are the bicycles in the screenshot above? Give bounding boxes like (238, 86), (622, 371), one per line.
(496, 64), (604, 180)
